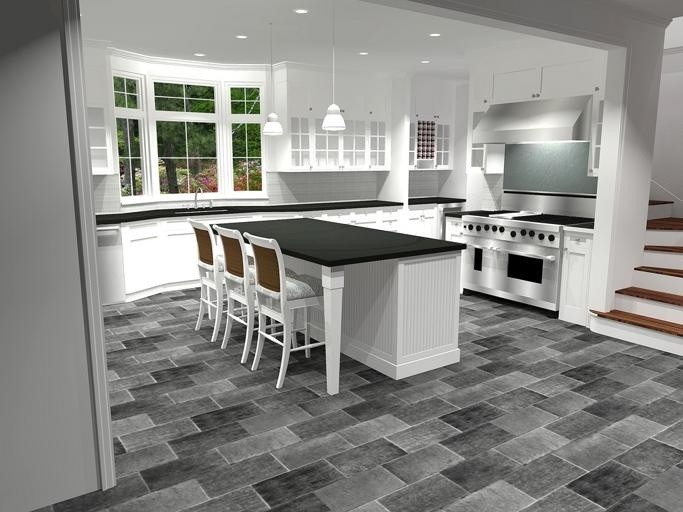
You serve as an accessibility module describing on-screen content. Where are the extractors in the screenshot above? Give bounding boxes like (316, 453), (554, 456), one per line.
(470, 95), (592, 144)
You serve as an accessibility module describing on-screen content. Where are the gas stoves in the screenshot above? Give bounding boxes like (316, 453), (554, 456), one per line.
(459, 208), (594, 248)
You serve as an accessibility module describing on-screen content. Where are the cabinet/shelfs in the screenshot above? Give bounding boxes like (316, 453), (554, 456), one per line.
(84, 39), (123, 176)
(264, 62), (314, 173)
(558, 226), (594, 330)
(587, 60), (607, 178)
(490, 60), (593, 104)
(367, 63), (393, 173)
(465, 71), (505, 176)
(407, 73), (455, 172)
(314, 64), (367, 174)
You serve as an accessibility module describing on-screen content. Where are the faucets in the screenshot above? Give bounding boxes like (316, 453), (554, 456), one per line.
(194, 187), (203, 210)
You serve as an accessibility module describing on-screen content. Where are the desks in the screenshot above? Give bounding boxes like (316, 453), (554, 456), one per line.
(200, 217), (466, 397)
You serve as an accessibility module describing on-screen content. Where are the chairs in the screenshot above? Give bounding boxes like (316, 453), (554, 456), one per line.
(183, 216), (326, 393)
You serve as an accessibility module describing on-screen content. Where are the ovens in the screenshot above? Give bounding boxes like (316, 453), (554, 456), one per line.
(460, 237), (561, 312)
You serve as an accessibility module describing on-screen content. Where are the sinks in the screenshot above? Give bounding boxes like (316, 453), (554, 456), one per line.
(174, 209), (229, 215)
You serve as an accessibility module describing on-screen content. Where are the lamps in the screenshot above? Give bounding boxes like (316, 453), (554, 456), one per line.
(321, 1), (346, 132)
(262, 22), (285, 138)
